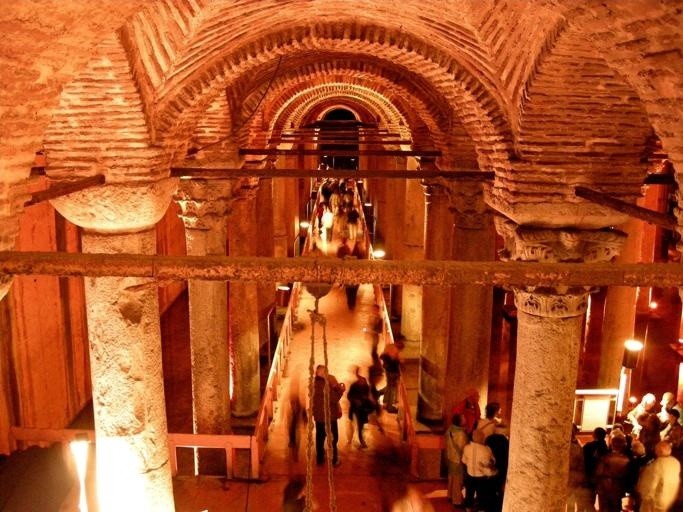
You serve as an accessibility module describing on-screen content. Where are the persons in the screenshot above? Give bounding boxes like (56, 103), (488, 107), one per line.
(281, 304), (431, 512)
(444, 392), (508, 512)
(310, 177), (368, 310)
(566, 392), (683, 512)
